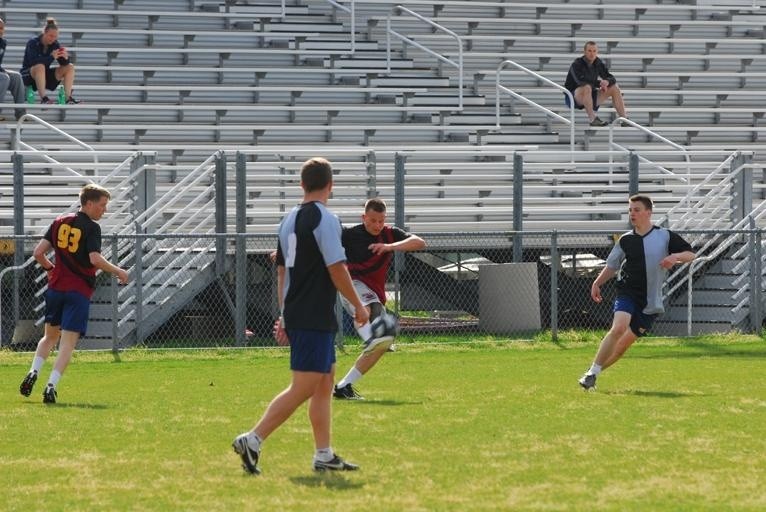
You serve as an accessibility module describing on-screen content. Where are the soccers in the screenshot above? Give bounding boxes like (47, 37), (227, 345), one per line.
(372, 315), (398, 339)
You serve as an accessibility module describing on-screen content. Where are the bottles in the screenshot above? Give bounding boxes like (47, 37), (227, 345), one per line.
(59, 86), (66, 103)
(27, 85), (35, 105)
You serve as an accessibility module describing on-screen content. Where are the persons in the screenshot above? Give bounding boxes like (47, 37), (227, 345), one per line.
(0, 18), (28, 123)
(563, 41), (632, 127)
(231, 156), (357, 474)
(21, 17), (81, 104)
(19, 184), (130, 403)
(270, 198), (426, 401)
(578, 196), (697, 389)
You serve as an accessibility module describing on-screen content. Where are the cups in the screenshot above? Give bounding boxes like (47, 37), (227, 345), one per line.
(244, 329), (254, 347)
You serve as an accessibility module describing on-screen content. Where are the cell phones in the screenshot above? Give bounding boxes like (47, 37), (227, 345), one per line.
(58, 47), (65, 57)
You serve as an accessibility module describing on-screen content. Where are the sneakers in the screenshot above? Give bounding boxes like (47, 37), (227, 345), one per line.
(579, 374), (596, 389)
(20, 370), (38, 397)
(232, 436), (260, 474)
(589, 116), (608, 125)
(42, 384), (57, 403)
(65, 96), (83, 103)
(620, 117), (634, 126)
(41, 96), (58, 110)
(314, 453), (359, 471)
(333, 383), (364, 399)
